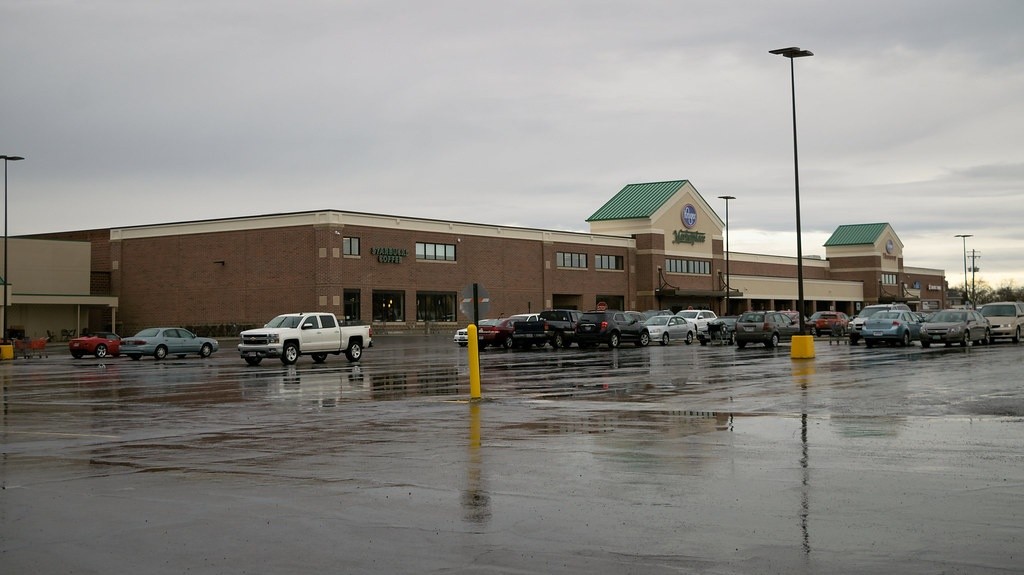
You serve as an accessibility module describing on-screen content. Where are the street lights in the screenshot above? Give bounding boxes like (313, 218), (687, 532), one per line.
(0, 154), (25, 360)
(955, 234), (972, 301)
(717, 196), (736, 317)
(768, 47), (816, 360)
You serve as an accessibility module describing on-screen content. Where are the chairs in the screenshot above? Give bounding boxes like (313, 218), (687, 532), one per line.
(47, 328), (88, 342)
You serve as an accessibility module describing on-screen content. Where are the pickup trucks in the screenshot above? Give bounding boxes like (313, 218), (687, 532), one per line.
(511, 308), (583, 350)
(237, 312), (373, 367)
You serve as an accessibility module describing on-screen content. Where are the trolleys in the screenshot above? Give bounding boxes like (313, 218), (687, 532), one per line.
(13, 336), (50, 360)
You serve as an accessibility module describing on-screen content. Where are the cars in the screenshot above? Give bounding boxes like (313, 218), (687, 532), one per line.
(919, 309), (991, 348)
(696, 315), (742, 346)
(778, 310), (858, 338)
(915, 309), (938, 321)
(642, 315), (693, 346)
(453, 313), (545, 351)
(118, 327), (219, 361)
(861, 309), (924, 349)
(68, 332), (122, 358)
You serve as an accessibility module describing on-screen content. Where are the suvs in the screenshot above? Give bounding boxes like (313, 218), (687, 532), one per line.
(624, 309), (673, 325)
(734, 311), (800, 348)
(847, 302), (911, 343)
(979, 301), (1024, 344)
(574, 309), (650, 350)
(674, 309), (718, 340)
